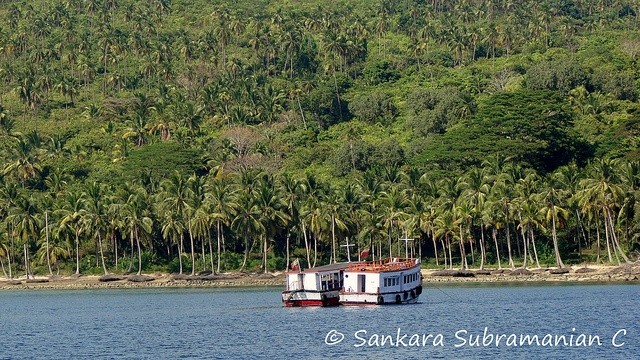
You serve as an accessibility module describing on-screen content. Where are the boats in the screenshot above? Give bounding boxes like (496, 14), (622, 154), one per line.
(282, 262), (366, 306)
(338, 258), (422, 305)
